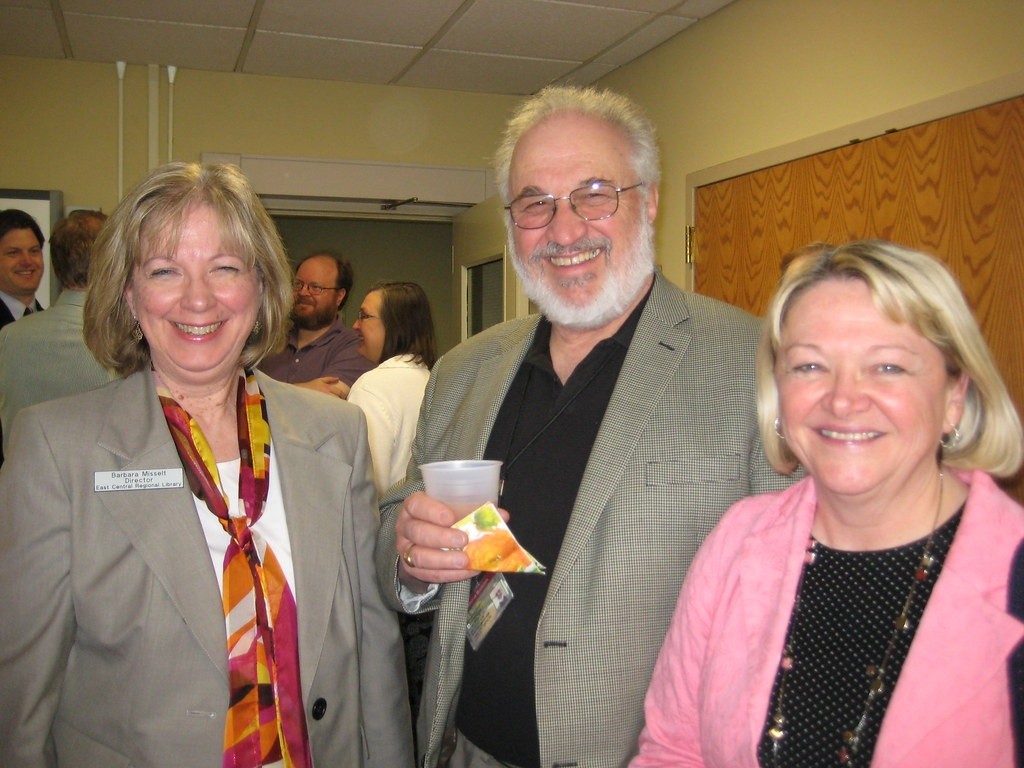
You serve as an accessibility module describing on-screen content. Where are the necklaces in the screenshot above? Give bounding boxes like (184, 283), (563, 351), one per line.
(768, 474), (944, 768)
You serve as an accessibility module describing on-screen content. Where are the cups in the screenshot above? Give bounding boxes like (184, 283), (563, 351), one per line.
(417, 459), (504, 524)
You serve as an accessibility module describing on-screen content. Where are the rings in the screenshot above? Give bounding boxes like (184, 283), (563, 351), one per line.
(403, 544), (416, 567)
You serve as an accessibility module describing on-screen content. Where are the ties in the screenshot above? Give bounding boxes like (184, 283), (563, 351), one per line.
(23, 306), (32, 318)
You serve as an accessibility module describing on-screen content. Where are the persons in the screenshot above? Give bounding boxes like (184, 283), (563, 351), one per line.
(625, 240), (1024, 768)
(375, 85), (771, 768)
(0, 160), (439, 768)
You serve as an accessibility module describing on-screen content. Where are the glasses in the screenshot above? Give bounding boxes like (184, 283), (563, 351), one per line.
(504, 183), (642, 230)
(357, 311), (383, 321)
(290, 278), (339, 296)
